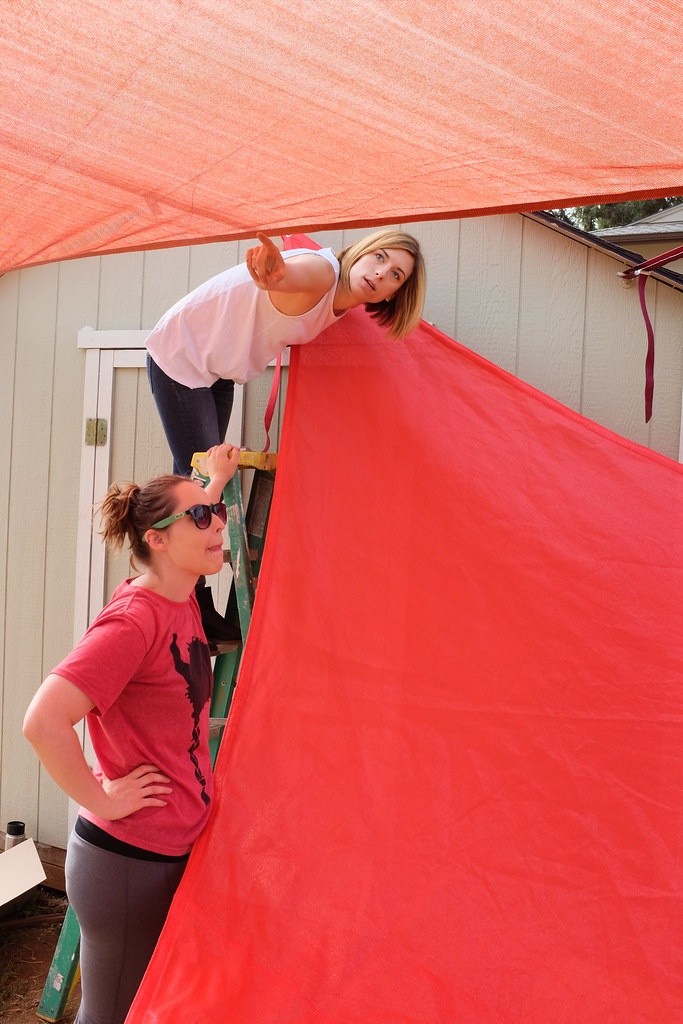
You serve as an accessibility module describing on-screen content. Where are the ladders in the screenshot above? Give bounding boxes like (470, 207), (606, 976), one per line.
(36, 443), (283, 1022)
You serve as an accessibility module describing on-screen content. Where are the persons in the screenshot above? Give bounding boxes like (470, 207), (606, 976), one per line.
(23, 443), (241, 1024)
(143, 230), (426, 644)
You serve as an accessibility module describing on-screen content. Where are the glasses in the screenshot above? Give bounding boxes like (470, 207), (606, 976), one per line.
(141, 503), (227, 543)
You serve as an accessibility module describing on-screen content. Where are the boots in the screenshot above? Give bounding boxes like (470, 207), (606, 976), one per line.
(195, 585), (243, 644)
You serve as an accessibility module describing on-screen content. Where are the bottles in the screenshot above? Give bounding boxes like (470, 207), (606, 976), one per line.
(4, 821), (25, 852)
